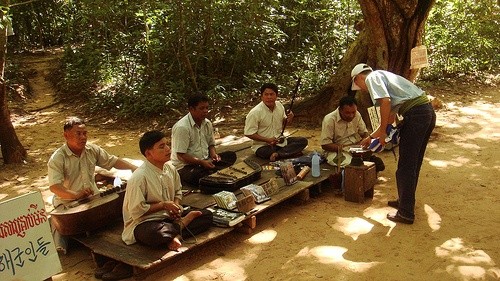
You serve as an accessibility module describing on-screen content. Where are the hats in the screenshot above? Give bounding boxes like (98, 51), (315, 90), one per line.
(351, 63), (373, 90)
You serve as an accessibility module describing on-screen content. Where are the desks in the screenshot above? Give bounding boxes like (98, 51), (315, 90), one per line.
(344, 161), (375, 202)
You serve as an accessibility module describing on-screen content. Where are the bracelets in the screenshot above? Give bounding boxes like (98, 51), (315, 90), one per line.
(369, 135), (375, 139)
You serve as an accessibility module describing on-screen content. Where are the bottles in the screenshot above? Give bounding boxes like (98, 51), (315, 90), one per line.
(312, 152), (320, 177)
(114, 175), (122, 186)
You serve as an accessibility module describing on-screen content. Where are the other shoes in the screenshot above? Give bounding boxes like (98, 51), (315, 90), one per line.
(387, 213), (414, 224)
(95, 260), (132, 281)
(388, 200), (399, 207)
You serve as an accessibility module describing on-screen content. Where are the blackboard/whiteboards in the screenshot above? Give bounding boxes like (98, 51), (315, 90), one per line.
(0, 190), (62, 281)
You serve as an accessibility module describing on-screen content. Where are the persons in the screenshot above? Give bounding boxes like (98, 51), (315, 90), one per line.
(121, 130), (202, 250)
(49, 117), (138, 255)
(171, 94), (237, 188)
(244, 84), (308, 161)
(322, 96), (385, 196)
(351, 64), (436, 224)
